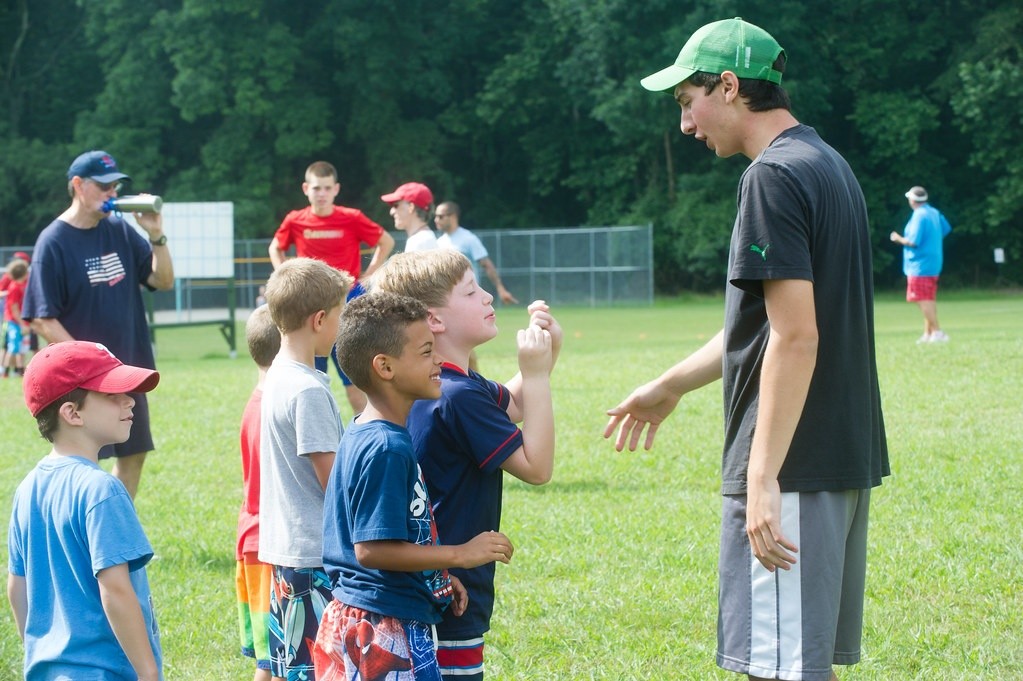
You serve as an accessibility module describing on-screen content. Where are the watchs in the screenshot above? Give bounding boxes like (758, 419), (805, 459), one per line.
(149, 236), (167, 246)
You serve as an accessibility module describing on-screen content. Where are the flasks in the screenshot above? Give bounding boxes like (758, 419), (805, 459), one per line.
(102, 195), (162, 214)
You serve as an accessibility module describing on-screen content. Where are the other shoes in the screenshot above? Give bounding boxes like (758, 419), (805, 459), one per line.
(917, 328), (950, 344)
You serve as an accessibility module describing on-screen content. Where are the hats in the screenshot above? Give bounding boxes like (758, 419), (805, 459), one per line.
(381, 182), (433, 210)
(67, 150), (132, 184)
(905, 186), (928, 201)
(639, 17), (788, 96)
(24, 341), (160, 418)
(6, 252), (30, 262)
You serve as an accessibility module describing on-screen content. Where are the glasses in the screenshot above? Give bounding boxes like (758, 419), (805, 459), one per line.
(389, 203), (398, 209)
(83, 177), (123, 190)
(432, 212), (453, 219)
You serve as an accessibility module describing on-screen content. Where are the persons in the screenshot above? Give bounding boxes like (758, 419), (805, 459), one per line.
(0, 251), (40, 377)
(890, 186), (952, 343)
(236, 161), (561, 681)
(21, 150), (175, 507)
(603, 17), (895, 681)
(8, 341), (163, 681)
